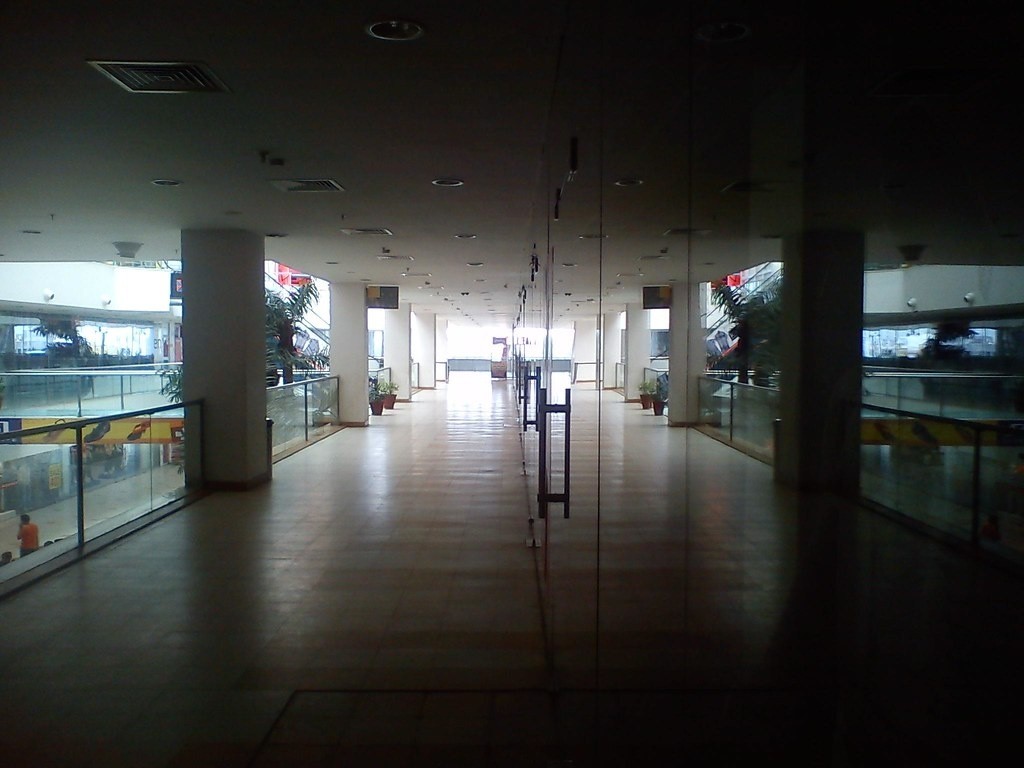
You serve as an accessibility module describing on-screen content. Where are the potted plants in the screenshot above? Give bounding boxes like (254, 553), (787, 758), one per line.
(382, 383), (399, 410)
(651, 382), (671, 415)
(639, 380), (654, 409)
(369, 383), (384, 416)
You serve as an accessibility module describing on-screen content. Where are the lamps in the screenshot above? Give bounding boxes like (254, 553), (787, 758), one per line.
(906, 298), (916, 307)
(102, 297), (113, 306)
(45, 288), (55, 301)
(963, 290), (978, 303)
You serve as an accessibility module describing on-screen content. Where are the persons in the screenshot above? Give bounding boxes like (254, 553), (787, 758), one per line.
(0, 514), (39, 568)
